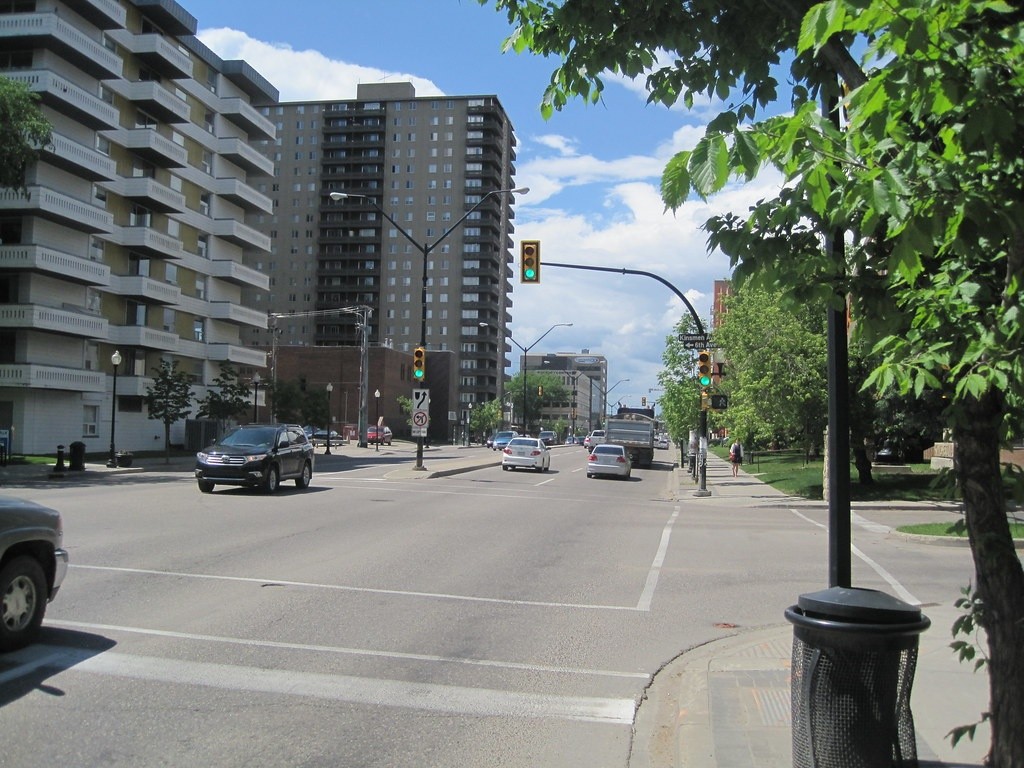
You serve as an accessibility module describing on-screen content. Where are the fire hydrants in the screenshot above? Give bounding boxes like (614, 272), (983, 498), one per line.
(53, 445), (67, 471)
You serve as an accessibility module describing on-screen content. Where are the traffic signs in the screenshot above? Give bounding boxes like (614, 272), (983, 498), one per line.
(684, 340), (722, 349)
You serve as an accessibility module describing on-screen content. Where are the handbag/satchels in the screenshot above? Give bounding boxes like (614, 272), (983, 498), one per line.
(730, 451), (735, 462)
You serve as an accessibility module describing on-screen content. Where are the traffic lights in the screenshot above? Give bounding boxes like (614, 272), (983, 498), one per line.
(413, 348), (425, 380)
(520, 239), (541, 284)
(699, 351), (712, 388)
(712, 395), (727, 409)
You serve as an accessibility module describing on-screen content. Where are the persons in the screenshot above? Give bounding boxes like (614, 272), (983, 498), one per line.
(730, 438), (743, 477)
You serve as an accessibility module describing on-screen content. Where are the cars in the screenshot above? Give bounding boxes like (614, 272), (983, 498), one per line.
(0, 493), (69, 651)
(303, 425), (343, 440)
(487, 426), (605, 451)
(654, 435), (672, 450)
(194, 423), (315, 493)
(367, 425), (393, 446)
(587, 444), (633, 481)
(502, 437), (552, 473)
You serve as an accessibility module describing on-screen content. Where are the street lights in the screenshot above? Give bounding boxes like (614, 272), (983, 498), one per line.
(324, 382), (334, 455)
(252, 373), (261, 423)
(479, 323), (574, 438)
(544, 361), (606, 444)
(105, 350), (122, 468)
(468, 402), (472, 446)
(330, 186), (531, 471)
(374, 389), (381, 452)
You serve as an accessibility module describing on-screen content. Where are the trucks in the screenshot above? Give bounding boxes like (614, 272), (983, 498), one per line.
(605, 407), (659, 467)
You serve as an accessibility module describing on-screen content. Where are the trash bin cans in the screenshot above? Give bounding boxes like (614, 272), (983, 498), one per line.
(69, 442), (86, 471)
(784, 585), (932, 768)
